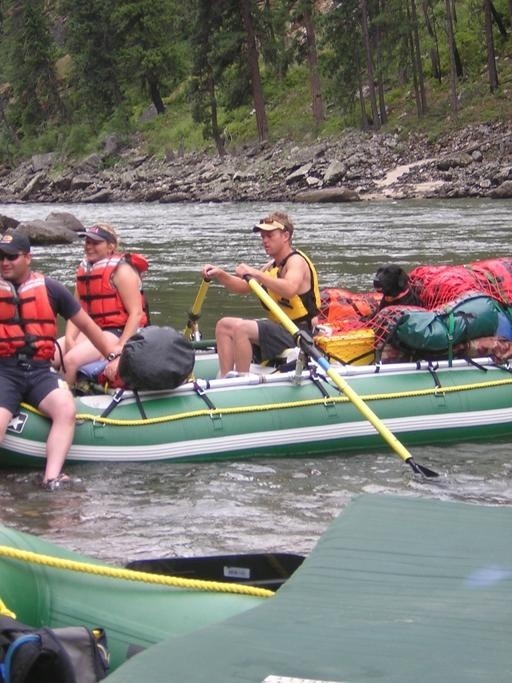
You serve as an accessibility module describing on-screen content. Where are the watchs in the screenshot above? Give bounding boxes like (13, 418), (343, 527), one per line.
(107, 351), (122, 362)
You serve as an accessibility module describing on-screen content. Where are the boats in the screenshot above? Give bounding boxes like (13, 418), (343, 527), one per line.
(0, 339), (512, 467)
(0, 521), (278, 678)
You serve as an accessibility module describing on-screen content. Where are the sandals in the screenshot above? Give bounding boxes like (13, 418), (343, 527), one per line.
(41, 473), (72, 492)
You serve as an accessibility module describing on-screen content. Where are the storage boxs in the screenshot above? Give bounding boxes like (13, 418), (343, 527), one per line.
(314, 329), (375, 367)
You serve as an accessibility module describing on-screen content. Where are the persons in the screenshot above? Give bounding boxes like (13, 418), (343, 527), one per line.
(201, 210), (322, 380)
(0, 230), (122, 494)
(45, 222), (151, 385)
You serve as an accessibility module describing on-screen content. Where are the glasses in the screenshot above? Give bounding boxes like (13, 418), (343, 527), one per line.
(260, 217), (274, 224)
(0, 252), (28, 261)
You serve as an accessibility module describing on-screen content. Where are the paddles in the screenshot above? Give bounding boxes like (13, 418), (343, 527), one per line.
(243, 274), (440, 479)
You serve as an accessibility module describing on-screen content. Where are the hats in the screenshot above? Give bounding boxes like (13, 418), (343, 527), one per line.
(0, 231), (30, 255)
(78, 227), (117, 243)
(252, 220), (288, 232)
